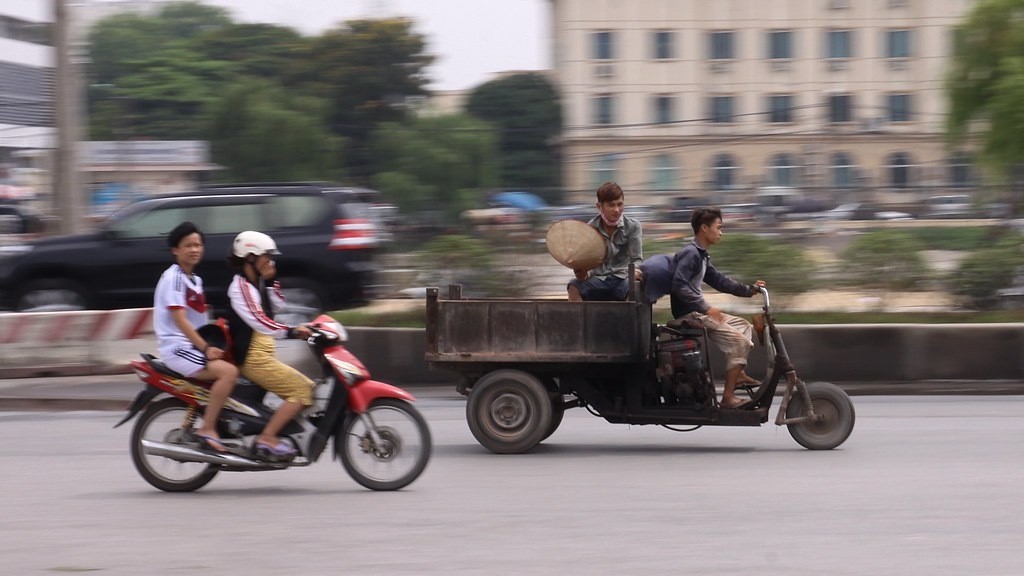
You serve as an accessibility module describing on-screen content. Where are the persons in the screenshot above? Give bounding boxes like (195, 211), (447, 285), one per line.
(669, 207), (766, 406)
(564, 182), (642, 304)
(152, 222), (239, 454)
(220, 231), (316, 457)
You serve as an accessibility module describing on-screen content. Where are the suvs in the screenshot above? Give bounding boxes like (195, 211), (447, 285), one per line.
(1, 178), (391, 314)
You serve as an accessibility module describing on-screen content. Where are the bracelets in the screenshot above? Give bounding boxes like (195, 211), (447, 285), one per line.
(203, 345), (210, 352)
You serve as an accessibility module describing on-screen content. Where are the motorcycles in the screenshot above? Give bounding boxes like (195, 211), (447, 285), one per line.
(114, 310), (433, 494)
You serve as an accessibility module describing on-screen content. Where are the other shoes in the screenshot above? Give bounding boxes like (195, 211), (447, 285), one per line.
(734, 378), (762, 389)
(719, 398), (753, 410)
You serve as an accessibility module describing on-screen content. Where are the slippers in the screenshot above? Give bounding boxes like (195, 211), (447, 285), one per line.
(255, 442), (299, 455)
(191, 431), (230, 452)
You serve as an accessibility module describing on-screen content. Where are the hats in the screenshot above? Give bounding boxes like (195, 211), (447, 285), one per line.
(545, 219), (609, 271)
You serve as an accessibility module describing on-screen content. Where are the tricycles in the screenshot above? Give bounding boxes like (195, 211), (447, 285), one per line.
(424, 250), (858, 453)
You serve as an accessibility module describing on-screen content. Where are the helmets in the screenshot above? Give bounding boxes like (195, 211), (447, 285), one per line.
(231, 230), (282, 258)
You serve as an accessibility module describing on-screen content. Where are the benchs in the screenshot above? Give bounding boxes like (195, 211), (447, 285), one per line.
(651, 300), (708, 368)
